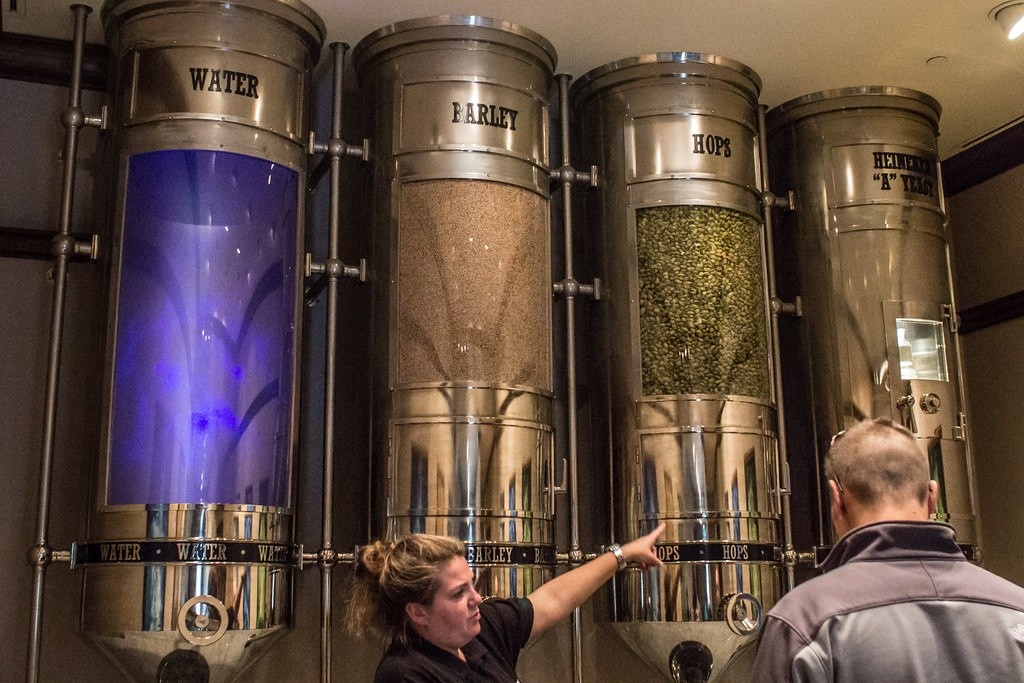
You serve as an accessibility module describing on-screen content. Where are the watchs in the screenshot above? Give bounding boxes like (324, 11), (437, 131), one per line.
(605, 542), (627, 570)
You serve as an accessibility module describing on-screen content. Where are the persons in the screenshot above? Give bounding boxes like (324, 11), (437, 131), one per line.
(345, 520), (667, 683)
(749, 417), (1024, 683)
